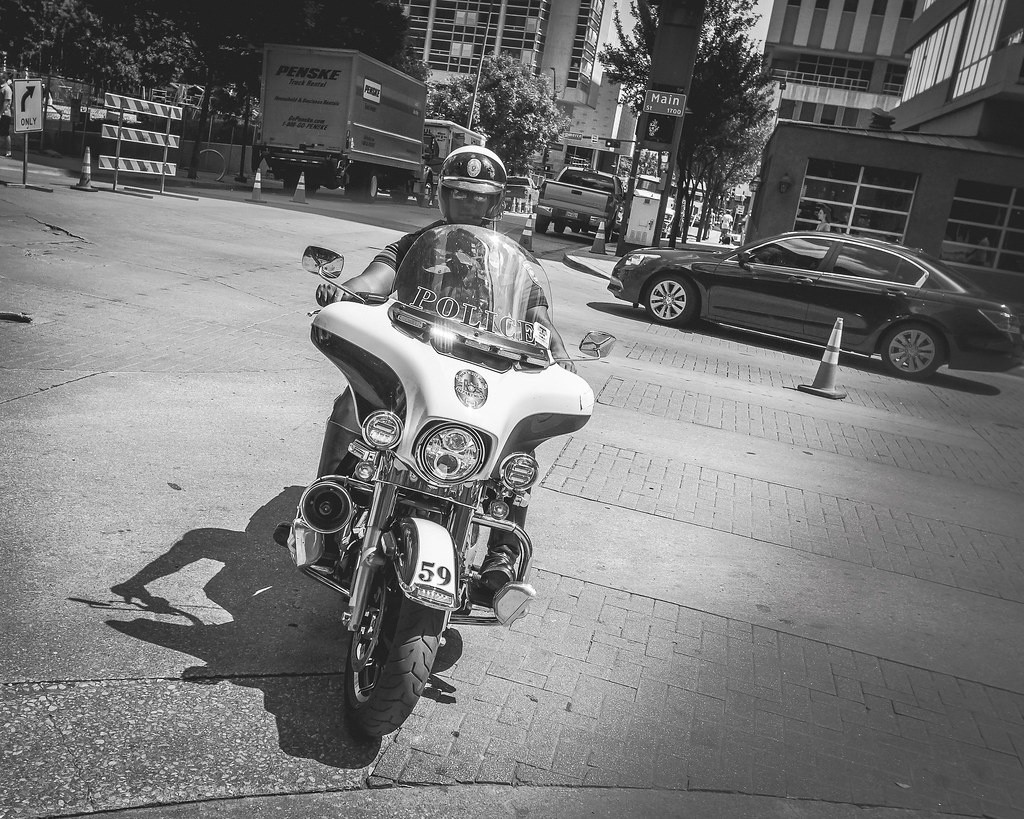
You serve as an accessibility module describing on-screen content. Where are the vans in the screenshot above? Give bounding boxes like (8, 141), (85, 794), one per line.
(635, 175), (685, 238)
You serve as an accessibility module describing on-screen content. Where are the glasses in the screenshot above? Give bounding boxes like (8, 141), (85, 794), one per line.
(448, 189), (491, 201)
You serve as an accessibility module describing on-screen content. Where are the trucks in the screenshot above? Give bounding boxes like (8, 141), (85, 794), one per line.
(259, 42), (436, 206)
(424, 118), (488, 206)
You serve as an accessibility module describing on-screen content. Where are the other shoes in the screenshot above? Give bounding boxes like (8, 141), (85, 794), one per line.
(479, 547), (518, 586)
(273, 522), (340, 565)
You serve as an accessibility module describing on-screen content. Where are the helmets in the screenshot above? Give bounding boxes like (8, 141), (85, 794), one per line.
(437, 145), (507, 221)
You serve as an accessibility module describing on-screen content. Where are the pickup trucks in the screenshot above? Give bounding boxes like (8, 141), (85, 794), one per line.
(532, 166), (625, 241)
(505, 176), (541, 212)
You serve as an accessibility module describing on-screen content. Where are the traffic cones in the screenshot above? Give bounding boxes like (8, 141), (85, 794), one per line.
(518, 215), (534, 252)
(589, 221), (607, 255)
(69, 145), (98, 192)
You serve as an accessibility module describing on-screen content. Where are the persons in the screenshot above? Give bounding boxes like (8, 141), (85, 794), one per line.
(708, 210), (748, 242)
(0, 70), (17, 157)
(271, 146), (580, 598)
(815, 203), (834, 232)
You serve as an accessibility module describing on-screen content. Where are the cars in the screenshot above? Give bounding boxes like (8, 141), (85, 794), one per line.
(607, 230), (1024, 380)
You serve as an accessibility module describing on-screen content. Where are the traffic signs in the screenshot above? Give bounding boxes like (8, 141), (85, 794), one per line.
(13, 78), (44, 133)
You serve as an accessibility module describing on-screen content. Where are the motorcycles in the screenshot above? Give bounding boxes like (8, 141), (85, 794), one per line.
(271, 244), (619, 736)
